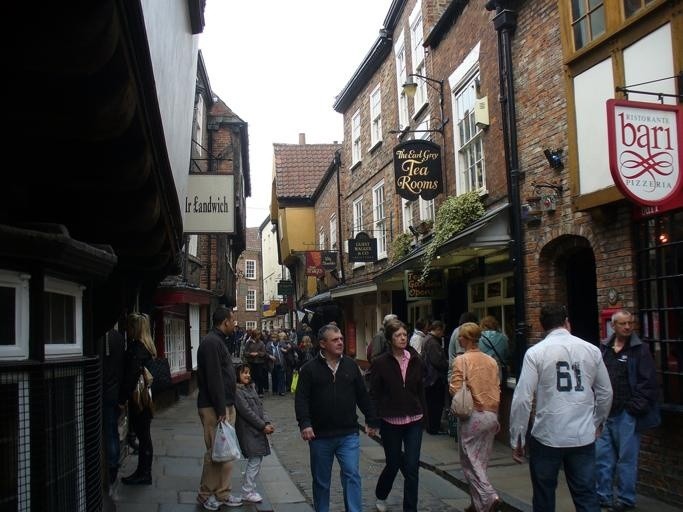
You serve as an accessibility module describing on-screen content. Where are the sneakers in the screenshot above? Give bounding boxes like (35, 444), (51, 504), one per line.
(281, 392), (285, 396)
(599, 504), (615, 512)
(259, 394), (264, 398)
(429, 430), (446, 435)
(122, 470), (152, 485)
(240, 493), (263, 503)
(490, 498), (505, 511)
(264, 388), (268, 392)
(376, 499), (387, 512)
(197, 494), (219, 510)
(216, 494), (243, 506)
(464, 504), (477, 511)
(608, 501), (635, 512)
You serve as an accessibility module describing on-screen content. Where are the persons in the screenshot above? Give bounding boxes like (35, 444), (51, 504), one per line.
(364, 302), (513, 447)
(294, 324), (380, 512)
(195, 304), (242, 511)
(121, 426), (140, 456)
(366, 320), (428, 511)
(232, 362), (276, 502)
(594, 309), (660, 510)
(221, 319), (320, 399)
(116, 311), (156, 486)
(508, 301), (614, 511)
(448, 322), (505, 511)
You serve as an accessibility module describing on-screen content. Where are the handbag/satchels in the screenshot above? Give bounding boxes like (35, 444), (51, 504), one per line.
(502, 365), (509, 389)
(450, 382), (473, 421)
(146, 358), (172, 394)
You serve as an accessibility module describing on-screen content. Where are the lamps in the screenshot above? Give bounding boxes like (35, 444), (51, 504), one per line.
(525, 180), (563, 214)
(402, 74), (445, 135)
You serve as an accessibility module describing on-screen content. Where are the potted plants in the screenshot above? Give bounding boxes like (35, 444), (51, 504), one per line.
(414, 217), (433, 235)
